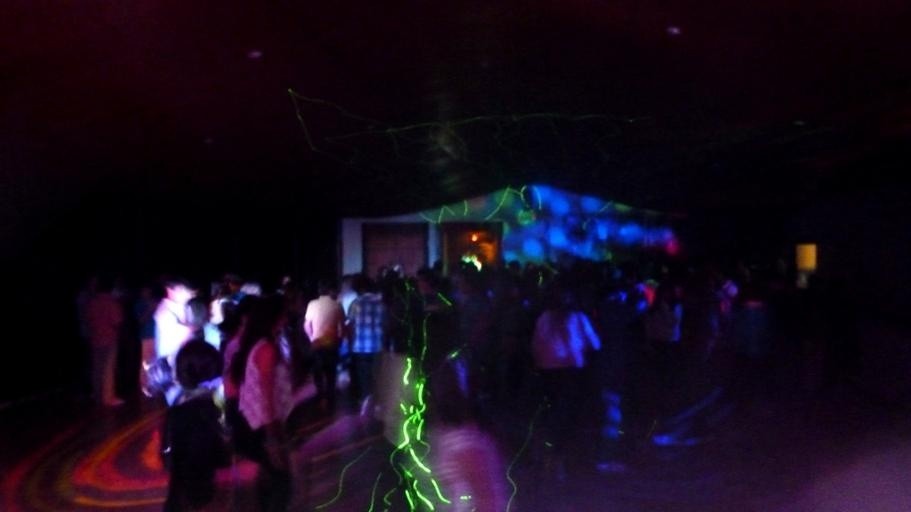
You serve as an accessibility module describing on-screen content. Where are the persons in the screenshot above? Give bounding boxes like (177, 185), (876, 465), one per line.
(72, 240), (766, 512)
